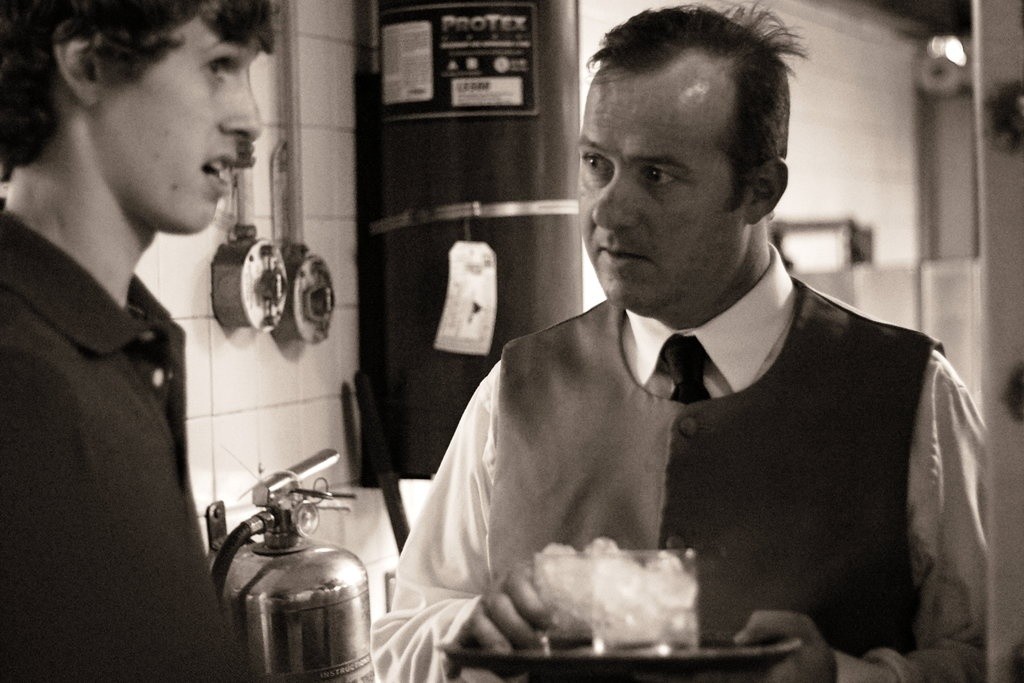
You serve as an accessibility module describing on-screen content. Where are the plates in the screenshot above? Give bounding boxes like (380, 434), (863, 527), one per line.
(443, 635), (802, 683)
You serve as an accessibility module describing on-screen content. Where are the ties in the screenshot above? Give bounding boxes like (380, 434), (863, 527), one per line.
(662, 334), (712, 403)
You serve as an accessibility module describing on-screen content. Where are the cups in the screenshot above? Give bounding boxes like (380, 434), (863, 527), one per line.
(535, 551), (624, 648)
(591, 551), (699, 654)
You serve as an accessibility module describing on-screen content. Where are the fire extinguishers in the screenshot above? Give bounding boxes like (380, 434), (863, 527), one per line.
(213, 447), (375, 683)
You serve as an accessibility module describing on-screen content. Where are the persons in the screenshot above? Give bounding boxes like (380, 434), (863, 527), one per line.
(0, 0), (275, 683)
(369, 4), (990, 683)
